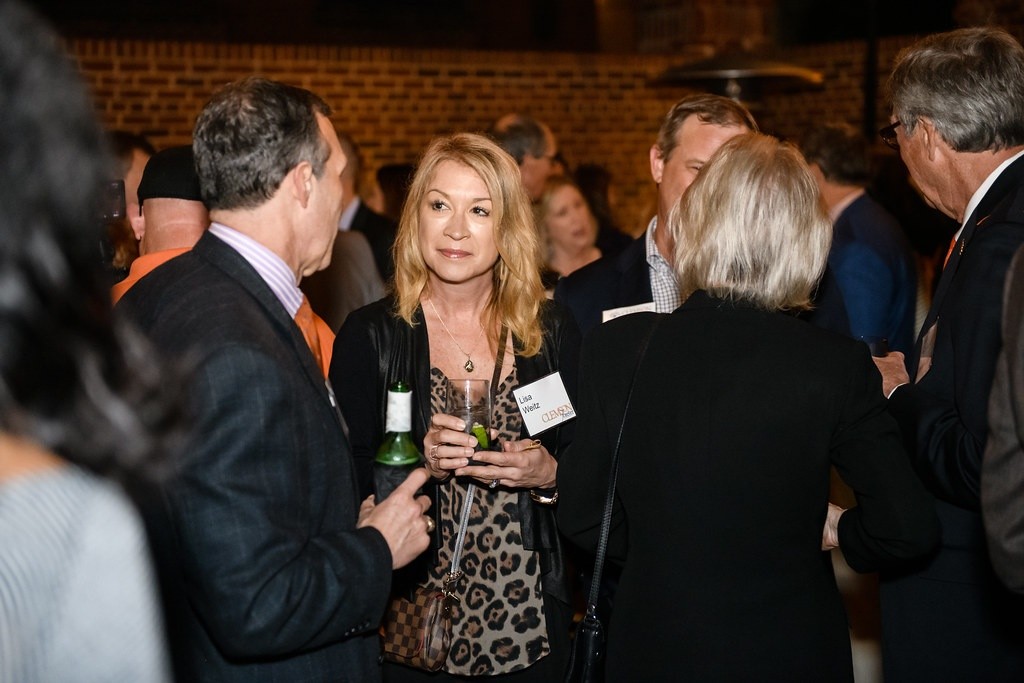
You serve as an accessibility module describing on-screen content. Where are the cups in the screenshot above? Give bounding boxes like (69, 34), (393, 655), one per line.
(855, 335), (889, 358)
(445, 379), (495, 475)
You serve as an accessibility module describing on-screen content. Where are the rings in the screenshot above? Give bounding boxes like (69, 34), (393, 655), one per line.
(430, 444), (440, 460)
(427, 516), (433, 531)
(489, 479), (500, 488)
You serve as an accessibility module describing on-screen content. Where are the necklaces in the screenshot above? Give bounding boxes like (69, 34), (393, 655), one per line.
(429, 299), (485, 372)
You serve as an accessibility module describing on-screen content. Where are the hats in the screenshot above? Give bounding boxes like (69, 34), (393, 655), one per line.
(137, 145), (202, 217)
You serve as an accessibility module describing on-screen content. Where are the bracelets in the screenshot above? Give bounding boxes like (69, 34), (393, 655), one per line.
(530, 487), (558, 504)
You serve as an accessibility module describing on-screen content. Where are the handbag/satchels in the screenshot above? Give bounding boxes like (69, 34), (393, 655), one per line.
(564, 616), (605, 683)
(377, 584), (456, 673)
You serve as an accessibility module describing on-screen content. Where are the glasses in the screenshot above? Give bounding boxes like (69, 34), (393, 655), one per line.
(880, 115), (918, 150)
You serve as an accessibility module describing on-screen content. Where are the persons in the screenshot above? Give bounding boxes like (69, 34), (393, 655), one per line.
(553, 94), (760, 336)
(870, 28), (1024, 683)
(114, 78), (435, 683)
(328, 133), (582, 683)
(980, 243), (1024, 595)
(111, 145), (336, 378)
(297, 134), (420, 334)
(795, 122), (963, 349)
(555, 133), (944, 683)
(103, 130), (158, 285)
(0, 0), (162, 683)
(489, 113), (635, 290)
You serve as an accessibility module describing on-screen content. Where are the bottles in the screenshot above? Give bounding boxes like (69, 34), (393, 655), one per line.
(371, 381), (427, 572)
